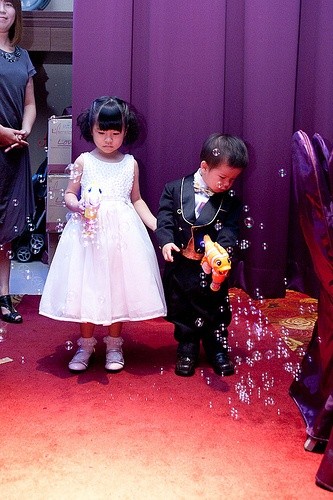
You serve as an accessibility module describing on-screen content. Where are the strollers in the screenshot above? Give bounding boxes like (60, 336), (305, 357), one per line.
(13, 105), (72, 263)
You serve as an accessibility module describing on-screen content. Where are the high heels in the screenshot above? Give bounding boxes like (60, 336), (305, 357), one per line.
(0, 294), (23, 323)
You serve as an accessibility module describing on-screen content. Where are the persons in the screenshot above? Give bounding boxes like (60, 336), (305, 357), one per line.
(39, 96), (158, 370)
(156, 134), (248, 376)
(0, 0), (37, 325)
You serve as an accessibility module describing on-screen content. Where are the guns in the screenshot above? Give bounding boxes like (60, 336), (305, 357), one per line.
(200, 234), (231, 291)
(83, 185), (102, 219)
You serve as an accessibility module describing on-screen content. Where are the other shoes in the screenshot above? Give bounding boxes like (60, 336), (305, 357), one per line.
(68, 337), (96, 373)
(102, 335), (125, 371)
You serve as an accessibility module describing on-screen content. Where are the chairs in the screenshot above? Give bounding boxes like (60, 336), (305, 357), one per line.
(287, 129), (333, 491)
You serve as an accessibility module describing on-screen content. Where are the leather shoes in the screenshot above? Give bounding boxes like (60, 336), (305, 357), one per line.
(175, 357), (195, 377)
(206, 353), (234, 376)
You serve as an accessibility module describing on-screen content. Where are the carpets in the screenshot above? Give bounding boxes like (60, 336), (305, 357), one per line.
(0, 295), (333, 500)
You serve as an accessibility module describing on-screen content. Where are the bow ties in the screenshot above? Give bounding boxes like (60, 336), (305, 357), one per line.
(193, 184), (215, 198)
